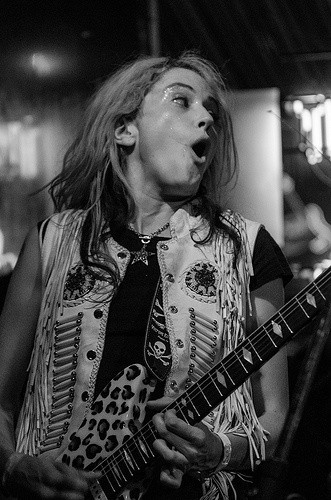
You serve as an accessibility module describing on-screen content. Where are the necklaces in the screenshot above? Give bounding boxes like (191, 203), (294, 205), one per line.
(128, 221), (170, 265)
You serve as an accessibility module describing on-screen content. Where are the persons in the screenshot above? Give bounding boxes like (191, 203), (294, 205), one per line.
(0, 53), (296, 500)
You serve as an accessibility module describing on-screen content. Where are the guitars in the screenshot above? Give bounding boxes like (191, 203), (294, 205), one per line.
(82, 267), (331, 500)
